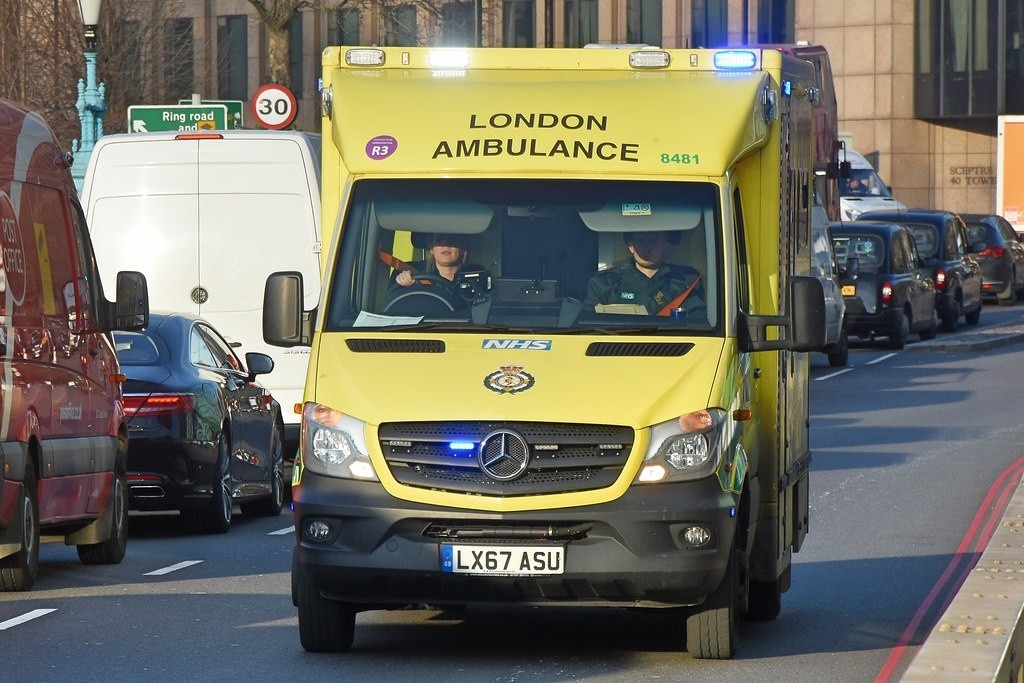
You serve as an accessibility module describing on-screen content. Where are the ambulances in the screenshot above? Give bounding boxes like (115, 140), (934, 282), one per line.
(263, 39), (830, 660)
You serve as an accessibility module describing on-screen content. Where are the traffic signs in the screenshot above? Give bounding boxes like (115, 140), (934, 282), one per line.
(129, 100), (243, 132)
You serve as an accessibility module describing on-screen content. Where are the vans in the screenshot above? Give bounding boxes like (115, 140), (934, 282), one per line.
(838, 149), (907, 223)
(1, 96), (148, 597)
(82, 128), (324, 446)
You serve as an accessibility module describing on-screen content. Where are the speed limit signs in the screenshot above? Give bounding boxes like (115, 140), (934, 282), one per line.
(255, 86), (297, 132)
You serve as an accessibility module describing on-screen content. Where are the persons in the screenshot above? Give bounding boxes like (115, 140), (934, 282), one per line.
(583, 229), (706, 318)
(387, 233), (491, 301)
(846, 175), (868, 194)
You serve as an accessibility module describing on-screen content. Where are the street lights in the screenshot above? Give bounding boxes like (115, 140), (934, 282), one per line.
(70, 1), (104, 155)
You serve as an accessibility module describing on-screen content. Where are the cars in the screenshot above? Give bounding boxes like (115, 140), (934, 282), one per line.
(949, 216), (1024, 307)
(826, 279), (847, 364)
(825, 222), (940, 349)
(856, 209), (983, 331)
(110, 316), (285, 532)
